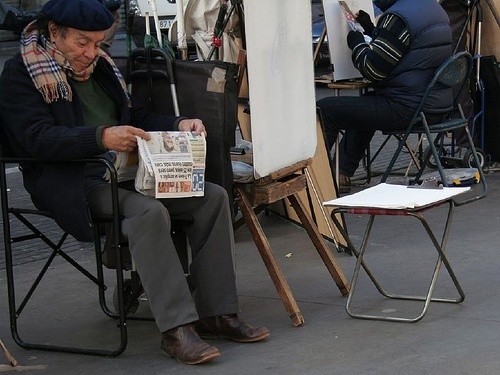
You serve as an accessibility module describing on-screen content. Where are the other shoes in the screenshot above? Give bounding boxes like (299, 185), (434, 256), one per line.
(339, 175), (351, 193)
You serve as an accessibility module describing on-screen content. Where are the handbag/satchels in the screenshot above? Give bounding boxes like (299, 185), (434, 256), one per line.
(131, 46), (243, 147)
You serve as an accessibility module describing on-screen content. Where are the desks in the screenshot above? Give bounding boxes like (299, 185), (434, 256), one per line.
(327, 81), (421, 186)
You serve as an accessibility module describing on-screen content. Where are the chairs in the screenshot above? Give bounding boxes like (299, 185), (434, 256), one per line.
(175, 56), (352, 326)
(1, 59), (180, 359)
(381, 50), (488, 206)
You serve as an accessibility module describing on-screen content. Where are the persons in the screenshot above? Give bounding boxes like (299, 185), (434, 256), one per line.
(316, 0), (452, 193)
(1, 0), (270, 365)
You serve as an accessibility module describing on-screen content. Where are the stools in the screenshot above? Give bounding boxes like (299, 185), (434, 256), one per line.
(324, 181), (473, 321)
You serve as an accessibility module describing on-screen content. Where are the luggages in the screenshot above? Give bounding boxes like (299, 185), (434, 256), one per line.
(134, 48), (235, 233)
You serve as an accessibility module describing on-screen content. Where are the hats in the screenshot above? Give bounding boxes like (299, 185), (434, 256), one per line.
(43, 0), (115, 31)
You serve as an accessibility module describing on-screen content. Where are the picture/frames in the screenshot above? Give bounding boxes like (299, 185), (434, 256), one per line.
(235, 102), (349, 250)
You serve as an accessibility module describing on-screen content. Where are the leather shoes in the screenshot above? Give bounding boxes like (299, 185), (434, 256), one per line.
(160, 323), (221, 364)
(194, 313), (270, 342)
